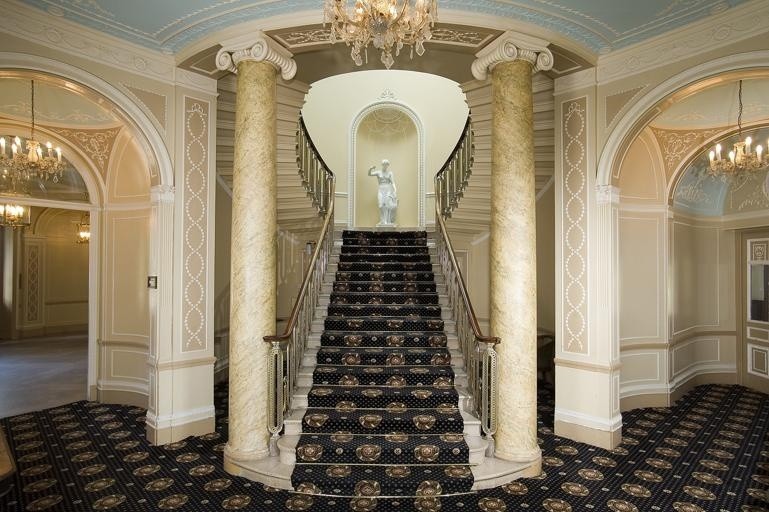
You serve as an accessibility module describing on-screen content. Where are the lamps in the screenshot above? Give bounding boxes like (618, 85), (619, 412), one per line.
(0, 80), (67, 189)
(707, 79), (768, 178)
(323, 1), (438, 69)
(1, 204), (31, 230)
(72, 212), (90, 244)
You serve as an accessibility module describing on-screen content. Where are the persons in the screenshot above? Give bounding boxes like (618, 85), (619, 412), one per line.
(368, 159), (397, 224)
